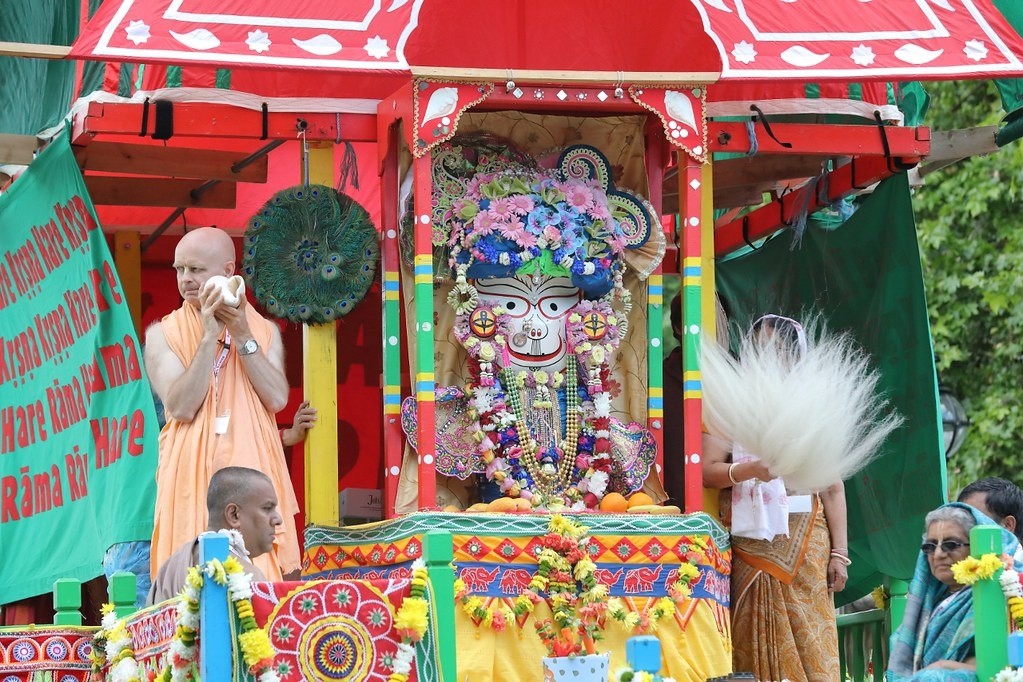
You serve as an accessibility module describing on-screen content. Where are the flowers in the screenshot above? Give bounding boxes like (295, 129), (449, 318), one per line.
(952, 551), (1023, 629)
(88, 556), (429, 682)
(871, 586), (885, 611)
(438, 163), (709, 644)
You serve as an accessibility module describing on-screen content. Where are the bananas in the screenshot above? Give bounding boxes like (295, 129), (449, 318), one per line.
(625, 505), (683, 514)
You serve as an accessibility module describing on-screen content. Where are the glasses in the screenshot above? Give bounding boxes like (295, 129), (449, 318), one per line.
(920, 542), (969, 553)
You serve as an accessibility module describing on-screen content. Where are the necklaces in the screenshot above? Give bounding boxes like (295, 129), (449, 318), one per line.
(502, 342), (578, 498)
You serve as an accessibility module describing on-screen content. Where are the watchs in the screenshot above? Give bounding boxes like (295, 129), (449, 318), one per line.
(238, 339), (258, 355)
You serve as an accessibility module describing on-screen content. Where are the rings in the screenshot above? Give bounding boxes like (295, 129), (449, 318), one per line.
(298, 417), (301, 422)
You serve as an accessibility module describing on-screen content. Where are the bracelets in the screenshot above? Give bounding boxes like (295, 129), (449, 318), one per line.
(831, 548), (852, 566)
(728, 462), (742, 485)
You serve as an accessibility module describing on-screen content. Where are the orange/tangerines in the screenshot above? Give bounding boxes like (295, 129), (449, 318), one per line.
(599, 492), (654, 513)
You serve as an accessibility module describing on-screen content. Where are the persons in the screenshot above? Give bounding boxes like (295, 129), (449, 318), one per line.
(278, 400), (318, 449)
(146, 466), (282, 609)
(701, 315), (852, 682)
(144, 227), (301, 584)
(886, 476), (1023, 682)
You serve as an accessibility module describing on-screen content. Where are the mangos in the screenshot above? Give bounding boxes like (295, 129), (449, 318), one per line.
(443, 497), (533, 514)
